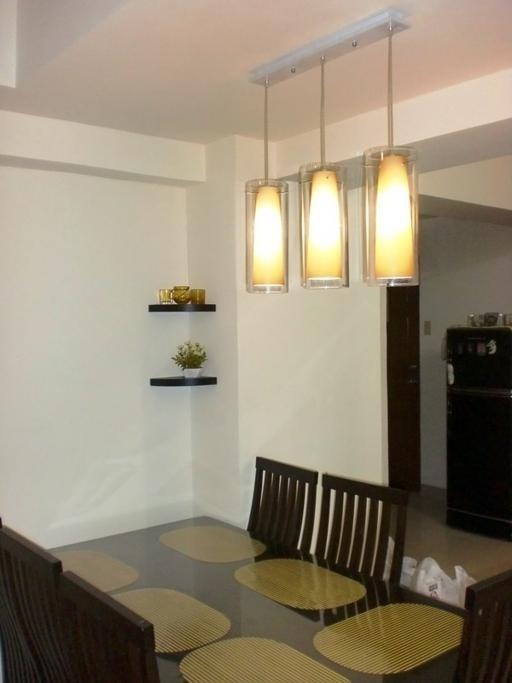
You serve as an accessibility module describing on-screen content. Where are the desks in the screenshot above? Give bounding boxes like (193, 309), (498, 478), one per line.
(43, 514), (472, 681)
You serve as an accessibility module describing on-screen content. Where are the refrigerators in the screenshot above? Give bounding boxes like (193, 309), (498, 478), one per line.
(444, 326), (512, 540)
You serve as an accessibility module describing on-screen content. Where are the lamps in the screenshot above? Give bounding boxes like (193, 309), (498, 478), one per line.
(244, 86), (289, 295)
(363, 37), (420, 288)
(298, 57), (348, 289)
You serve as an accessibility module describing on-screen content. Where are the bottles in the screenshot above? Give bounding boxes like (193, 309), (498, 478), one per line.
(171, 285), (191, 305)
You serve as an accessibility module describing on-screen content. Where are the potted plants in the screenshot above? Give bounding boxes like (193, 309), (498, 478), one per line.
(170, 342), (209, 378)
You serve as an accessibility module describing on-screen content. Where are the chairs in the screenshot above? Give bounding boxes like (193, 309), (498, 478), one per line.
(456, 572), (511, 681)
(312, 471), (410, 585)
(56, 570), (160, 683)
(1, 522), (65, 681)
(247, 457), (319, 555)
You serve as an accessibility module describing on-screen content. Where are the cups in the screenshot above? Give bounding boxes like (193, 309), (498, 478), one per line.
(158, 289), (173, 304)
(190, 288), (205, 305)
